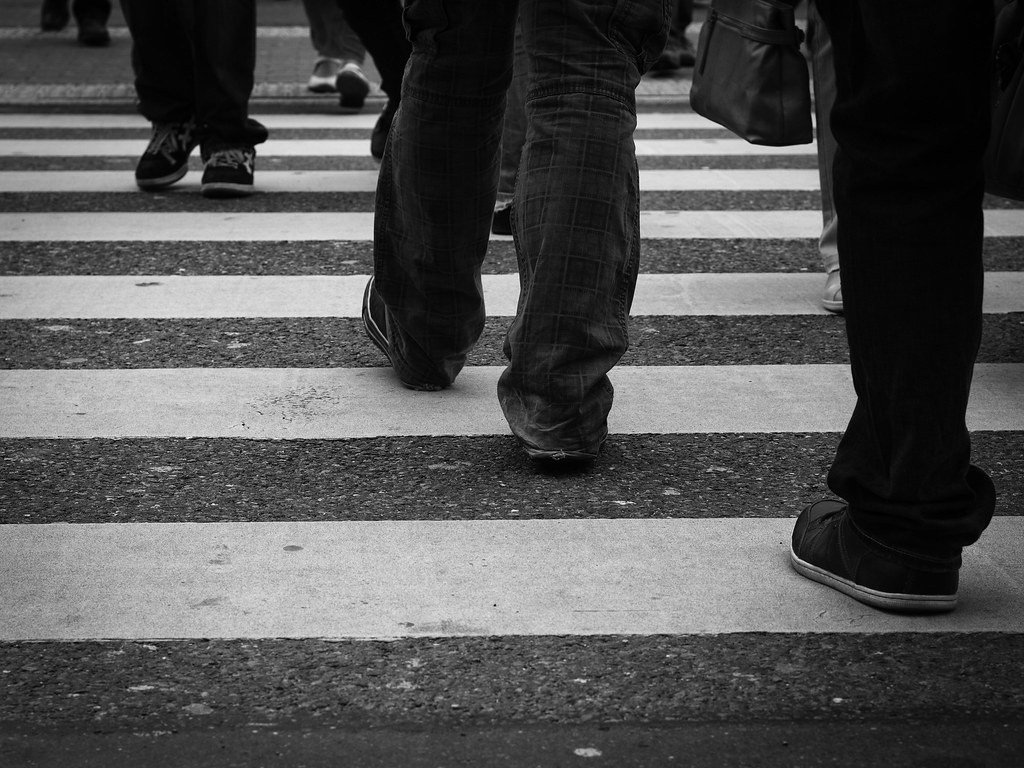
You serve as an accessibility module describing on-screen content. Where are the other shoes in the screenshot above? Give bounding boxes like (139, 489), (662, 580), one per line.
(41, 0), (70, 30)
(521, 427), (608, 475)
(308, 55), (343, 93)
(489, 199), (515, 242)
(370, 100), (397, 160)
(363, 274), (443, 391)
(651, 37), (695, 71)
(79, 16), (111, 47)
(336, 59), (371, 109)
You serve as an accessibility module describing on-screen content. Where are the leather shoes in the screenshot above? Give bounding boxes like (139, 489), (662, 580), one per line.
(821, 270), (844, 313)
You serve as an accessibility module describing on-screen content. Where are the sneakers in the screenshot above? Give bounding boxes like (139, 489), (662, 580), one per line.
(135, 107), (199, 189)
(200, 144), (256, 198)
(790, 498), (960, 611)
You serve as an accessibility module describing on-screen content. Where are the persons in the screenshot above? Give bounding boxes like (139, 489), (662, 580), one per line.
(334, 0), (1024, 611)
(302, 0), (370, 107)
(40, 0), (114, 47)
(119, 0), (269, 195)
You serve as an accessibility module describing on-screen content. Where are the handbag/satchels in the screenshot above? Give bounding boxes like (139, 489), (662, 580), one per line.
(689, 0), (814, 147)
(982, 0), (1024, 203)
